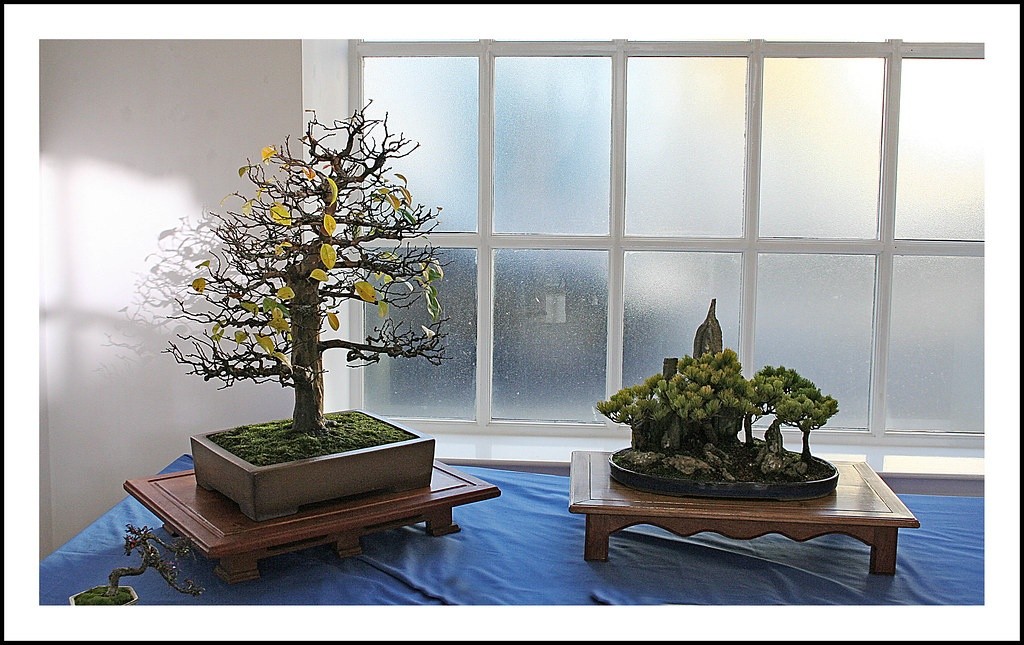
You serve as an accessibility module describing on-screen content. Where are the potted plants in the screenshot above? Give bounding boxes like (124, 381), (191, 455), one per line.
(71, 524), (205, 608)
(594, 350), (841, 497)
(164, 107), (453, 517)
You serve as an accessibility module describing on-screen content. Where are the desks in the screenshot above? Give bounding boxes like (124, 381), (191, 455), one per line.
(40, 448), (984, 605)
